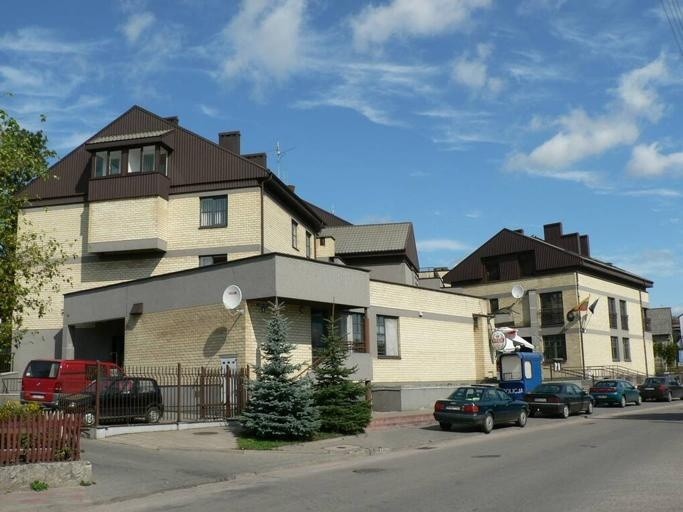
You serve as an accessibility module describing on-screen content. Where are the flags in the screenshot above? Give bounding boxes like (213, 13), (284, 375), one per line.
(589, 299), (600, 315)
(571, 296), (589, 312)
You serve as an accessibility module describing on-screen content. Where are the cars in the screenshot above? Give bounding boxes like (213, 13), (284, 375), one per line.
(433, 374), (683, 433)
(59, 375), (165, 426)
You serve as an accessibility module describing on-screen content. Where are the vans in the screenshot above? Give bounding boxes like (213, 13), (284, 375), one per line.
(20, 357), (128, 409)
